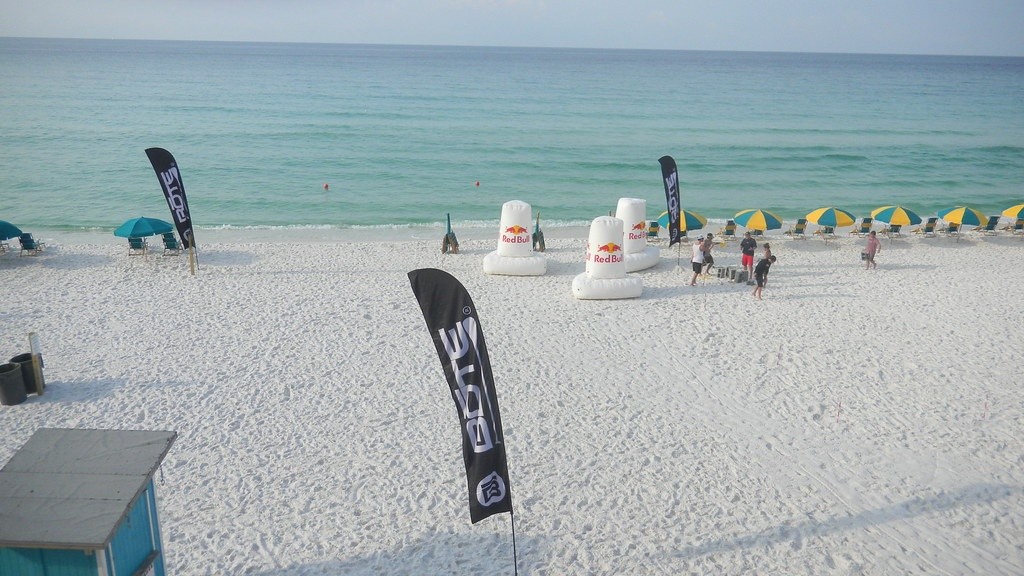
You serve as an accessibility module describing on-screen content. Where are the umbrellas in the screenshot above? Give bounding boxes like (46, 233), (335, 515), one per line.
(114, 216), (175, 251)
(655, 203), (1024, 242)
(0, 219), (23, 242)
(534, 211), (540, 251)
(446, 213), (450, 253)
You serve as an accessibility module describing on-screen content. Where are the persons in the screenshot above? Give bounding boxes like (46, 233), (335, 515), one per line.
(740, 232), (757, 281)
(698, 233), (723, 277)
(690, 234), (716, 286)
(762, 242), (771, 288)
(864, 230), (881, 270)
(751, 255), (776, 299)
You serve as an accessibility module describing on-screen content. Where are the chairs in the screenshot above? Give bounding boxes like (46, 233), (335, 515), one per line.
(910, 217), (939, 238)
(128, 238), (146, 256)
(847, 217), (874, 238)
(722, 221), (736, 238)
(998, 218), (1024, 236)
(783, 218), (808, 240)
(938, 222), (961, 238)
(646, 222), (660, 243)
(750, 230), (765, 238)
(18, 233), (37, 257)
(680, 231), (689, 243)
(161, 232), (180, 257)
(877, 224), (902, 239)
(813, 225), (837, 238)
(970, 215), (1001, 236)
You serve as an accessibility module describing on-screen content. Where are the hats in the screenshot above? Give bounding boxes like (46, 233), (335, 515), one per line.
(697, 235), (704, 240)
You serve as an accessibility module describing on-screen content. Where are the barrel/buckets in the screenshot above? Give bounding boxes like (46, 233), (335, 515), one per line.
(8, 352), (46, 394)
(0, 363), (28, 406)
